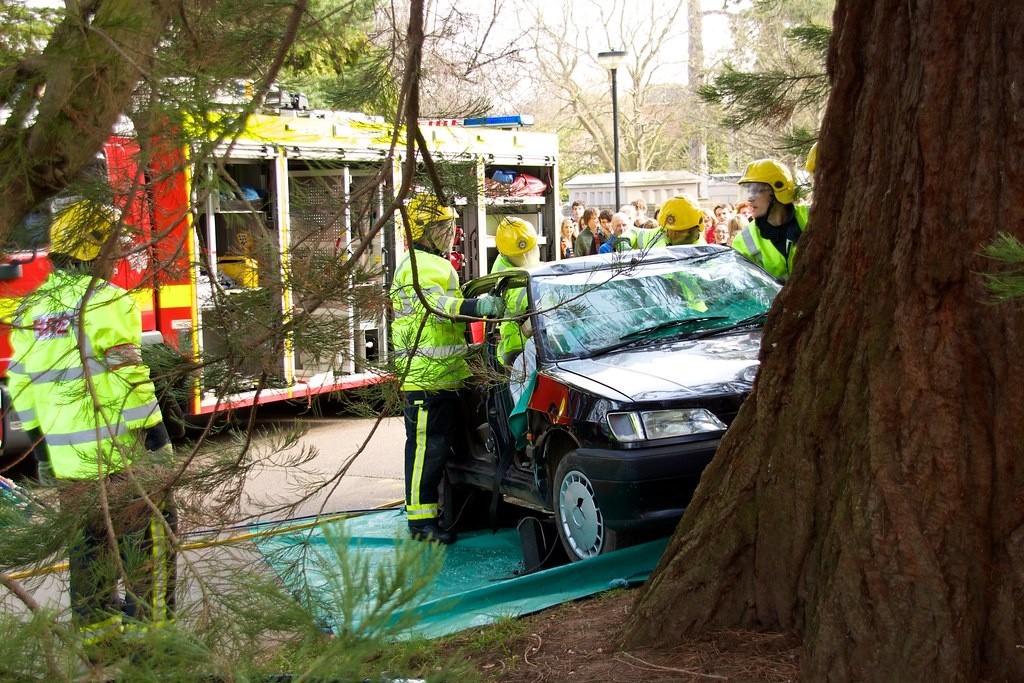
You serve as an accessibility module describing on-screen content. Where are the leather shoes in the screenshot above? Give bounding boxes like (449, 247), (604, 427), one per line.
(411, 523), (457, 545)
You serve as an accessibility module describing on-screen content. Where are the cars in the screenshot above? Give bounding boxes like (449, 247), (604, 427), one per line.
(424, 240), (785, 568)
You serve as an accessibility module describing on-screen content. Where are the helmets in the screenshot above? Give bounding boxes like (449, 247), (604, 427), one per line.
(737, 160), (795, 204)
(49, 200), (134, 261)
(496, 216), (536, 255)
(805, 141), (818, 172)
(404, 192), (460, 240)
(657, 196), (704, 232)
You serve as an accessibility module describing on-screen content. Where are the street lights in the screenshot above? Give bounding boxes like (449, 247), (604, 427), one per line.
(596, 47), (629, 215)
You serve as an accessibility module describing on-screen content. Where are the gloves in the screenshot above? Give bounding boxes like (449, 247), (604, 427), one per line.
(522, 315), (547, 338)
(39, 459), (58, 487)
(474, 292), (503, 316)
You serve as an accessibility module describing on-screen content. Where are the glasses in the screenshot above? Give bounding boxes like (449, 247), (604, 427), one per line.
(714, 231), (728, 235)
(745, 189), (771, 199)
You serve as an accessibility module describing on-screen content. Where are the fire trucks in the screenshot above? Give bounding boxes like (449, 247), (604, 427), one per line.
(0, 68), (563, 475)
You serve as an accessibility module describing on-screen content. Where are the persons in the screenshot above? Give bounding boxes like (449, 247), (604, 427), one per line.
(487, 216), (539, 369)
(5, 201), (175, 681)
(389, 194), (505, 544)
(731, 159), (810, 280)
(559, 142), (818, 312)
